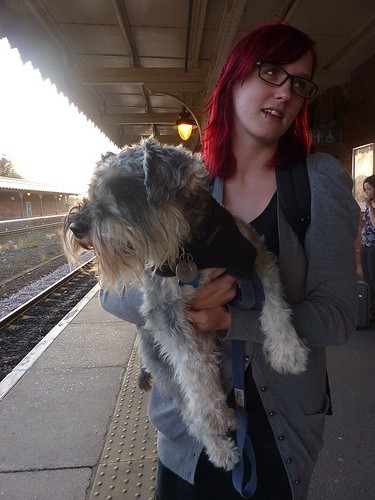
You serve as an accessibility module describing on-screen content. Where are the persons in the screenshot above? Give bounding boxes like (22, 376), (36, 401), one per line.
(360, 174), (375, 323)
(100, 20), (361, 500)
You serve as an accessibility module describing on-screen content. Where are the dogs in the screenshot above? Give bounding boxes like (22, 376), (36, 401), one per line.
(61, 133), (314, 473)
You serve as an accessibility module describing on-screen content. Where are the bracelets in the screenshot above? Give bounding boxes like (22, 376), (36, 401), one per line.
(368, 208), (374, 210)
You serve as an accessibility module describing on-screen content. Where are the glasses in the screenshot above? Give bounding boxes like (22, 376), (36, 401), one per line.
(254, 60), (318, 100)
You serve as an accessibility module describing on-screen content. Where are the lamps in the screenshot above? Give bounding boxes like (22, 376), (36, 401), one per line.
(172, 104), (198, 141)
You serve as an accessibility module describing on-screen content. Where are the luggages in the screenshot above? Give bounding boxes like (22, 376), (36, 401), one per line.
(354, 280), (371, 329)
